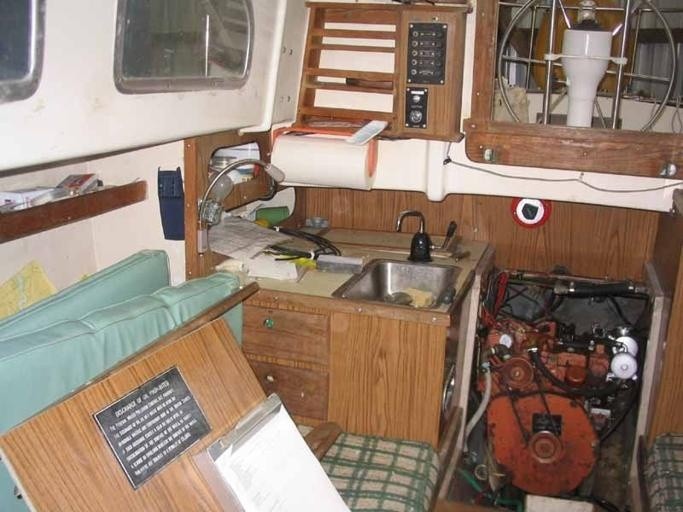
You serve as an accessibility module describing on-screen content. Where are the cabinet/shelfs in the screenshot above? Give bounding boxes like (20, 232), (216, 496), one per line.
(241, 301), (330, 420)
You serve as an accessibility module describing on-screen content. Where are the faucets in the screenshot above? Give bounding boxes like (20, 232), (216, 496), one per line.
(393, 210), (434, 262)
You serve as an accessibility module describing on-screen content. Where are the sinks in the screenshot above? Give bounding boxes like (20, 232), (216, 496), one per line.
(331, 257), (463, 310)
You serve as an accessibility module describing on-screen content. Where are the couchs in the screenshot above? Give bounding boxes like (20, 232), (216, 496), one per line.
(1, 248), (433, 512)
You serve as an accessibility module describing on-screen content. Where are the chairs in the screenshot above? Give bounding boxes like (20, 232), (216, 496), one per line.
(629, 214), (682, 510)
